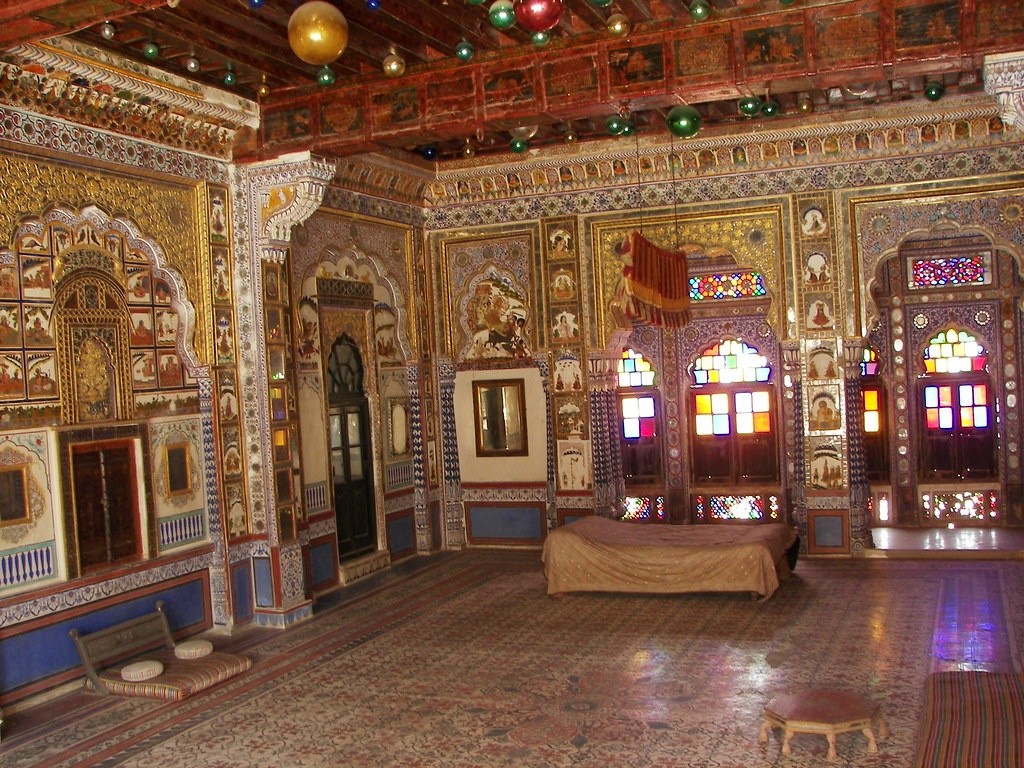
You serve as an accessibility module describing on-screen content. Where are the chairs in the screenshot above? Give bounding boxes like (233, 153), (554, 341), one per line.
(68, 599), (252, 701)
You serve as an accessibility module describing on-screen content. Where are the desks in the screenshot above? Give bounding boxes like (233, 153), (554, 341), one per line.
(758, 689), (888, 760)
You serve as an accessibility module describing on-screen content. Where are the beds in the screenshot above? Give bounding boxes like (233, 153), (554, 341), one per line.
(540, 516), (801, 601)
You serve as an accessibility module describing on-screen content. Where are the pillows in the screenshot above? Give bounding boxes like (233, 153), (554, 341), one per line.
(121, 661), (164, 681)
(174, 639), (213, 659)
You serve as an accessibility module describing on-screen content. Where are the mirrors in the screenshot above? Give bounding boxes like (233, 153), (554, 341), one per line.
(162, 441), (193, 497)
(261, 262), (296, 546)
(471, 378), (528, 458)
(0, 463), (33, 528)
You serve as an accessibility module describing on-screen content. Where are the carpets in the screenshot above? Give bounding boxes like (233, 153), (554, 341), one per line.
(910, 672), (1024, 768)
